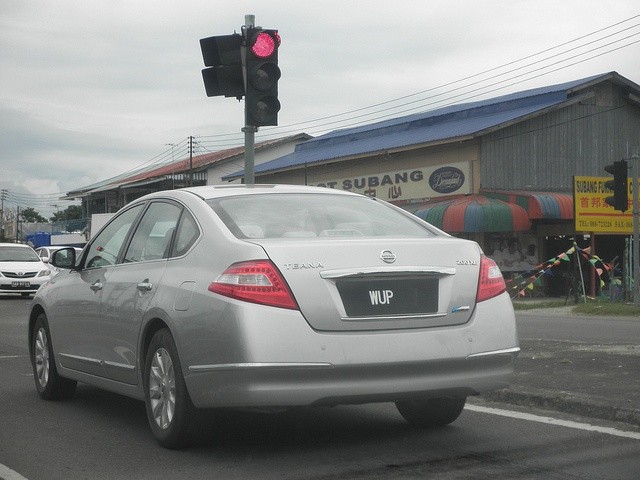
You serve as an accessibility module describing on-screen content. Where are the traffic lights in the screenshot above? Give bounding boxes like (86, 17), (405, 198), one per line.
(247, 30), (281, 127)
(605, 160), (627, 212)
(200, 35), (242, 96)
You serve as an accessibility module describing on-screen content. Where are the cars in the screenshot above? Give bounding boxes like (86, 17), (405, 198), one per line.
(27, 184), (521, 449)
(35, 244), (82, 266)
(0, 241), (51, 301)
